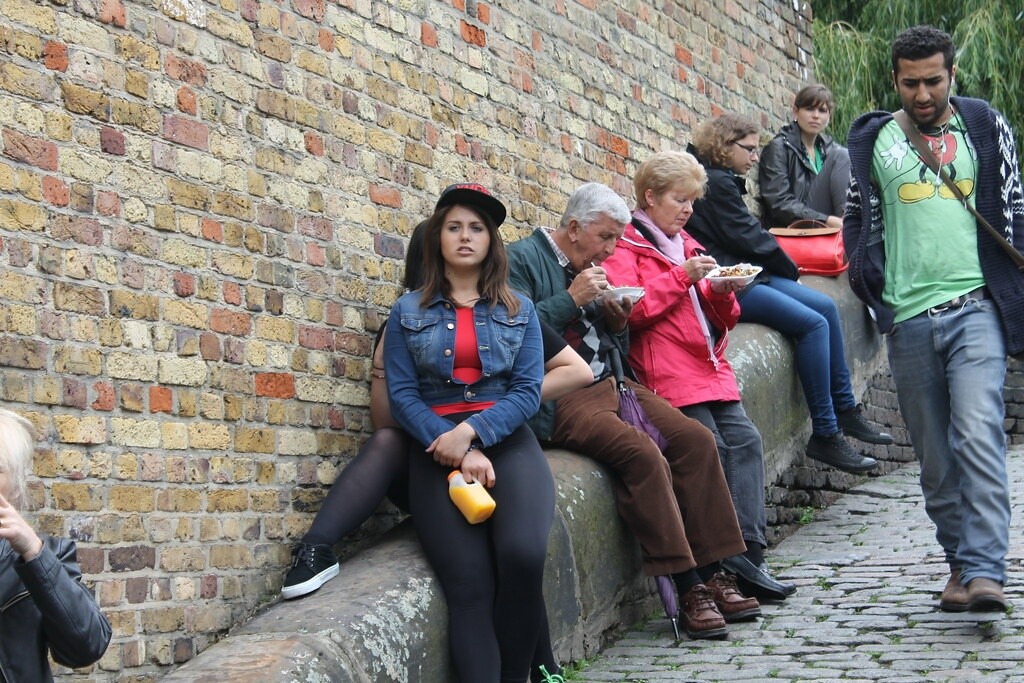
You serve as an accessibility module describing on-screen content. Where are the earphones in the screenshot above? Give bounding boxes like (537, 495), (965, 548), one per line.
(951, 77), (954, 87)
(935, 171), (941, 186)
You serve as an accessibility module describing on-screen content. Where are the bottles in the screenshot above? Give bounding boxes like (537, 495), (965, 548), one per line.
(447, 471), (495, 524)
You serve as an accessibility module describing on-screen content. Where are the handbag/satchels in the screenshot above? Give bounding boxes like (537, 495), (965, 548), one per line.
(768, 219), (850, 277)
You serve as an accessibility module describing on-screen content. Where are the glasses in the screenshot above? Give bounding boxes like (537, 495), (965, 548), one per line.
(731, 140), (759, 157)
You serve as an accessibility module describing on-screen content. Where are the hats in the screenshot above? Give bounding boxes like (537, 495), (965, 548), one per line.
(436, 183), (506, 228)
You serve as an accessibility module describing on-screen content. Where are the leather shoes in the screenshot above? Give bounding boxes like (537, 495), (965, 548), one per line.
(707, 571), (762, 620)
(755, 561), (797, 595)
(721, 552), (789, 602)
(679, 583), (729, 639)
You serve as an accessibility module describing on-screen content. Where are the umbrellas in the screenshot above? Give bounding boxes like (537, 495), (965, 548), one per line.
(611, 348), (681, 641)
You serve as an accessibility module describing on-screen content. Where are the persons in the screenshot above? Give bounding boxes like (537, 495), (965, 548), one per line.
(598, 150), (797, 601)
(382, 183), (556, 683)
(843, 25), (1024, 613)
(0, 408), (114, 683)
(279, 220), (593, 602)
(686, 111), (893, 473)
(759, 82), (851, 231)
(503, 182), (764, 639)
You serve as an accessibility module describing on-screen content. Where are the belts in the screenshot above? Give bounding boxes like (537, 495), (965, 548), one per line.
(928, 285), (994, 313)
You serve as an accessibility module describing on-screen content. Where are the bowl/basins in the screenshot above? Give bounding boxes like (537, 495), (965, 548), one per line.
(706, 266), (762, 286)
(595, 287), (644, 306)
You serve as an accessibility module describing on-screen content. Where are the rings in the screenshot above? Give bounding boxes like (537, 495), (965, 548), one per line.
(703, 269), (708, 276)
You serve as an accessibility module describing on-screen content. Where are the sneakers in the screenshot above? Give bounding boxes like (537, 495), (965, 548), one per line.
(968, 579), (1007, 612)
(281, 541), (339, 599)
(803, 428), (878, 472)
(940, 569), (970, 611)
(835, 402), (895, 445)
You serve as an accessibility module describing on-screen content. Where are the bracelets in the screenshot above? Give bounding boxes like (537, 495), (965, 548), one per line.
(371, 372), (386, 379)
(372, 361), (386, 370)
(465, 444), (479, 455)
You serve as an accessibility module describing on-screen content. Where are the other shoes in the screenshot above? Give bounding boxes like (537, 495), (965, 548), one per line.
(530, 663), (565, 683)
